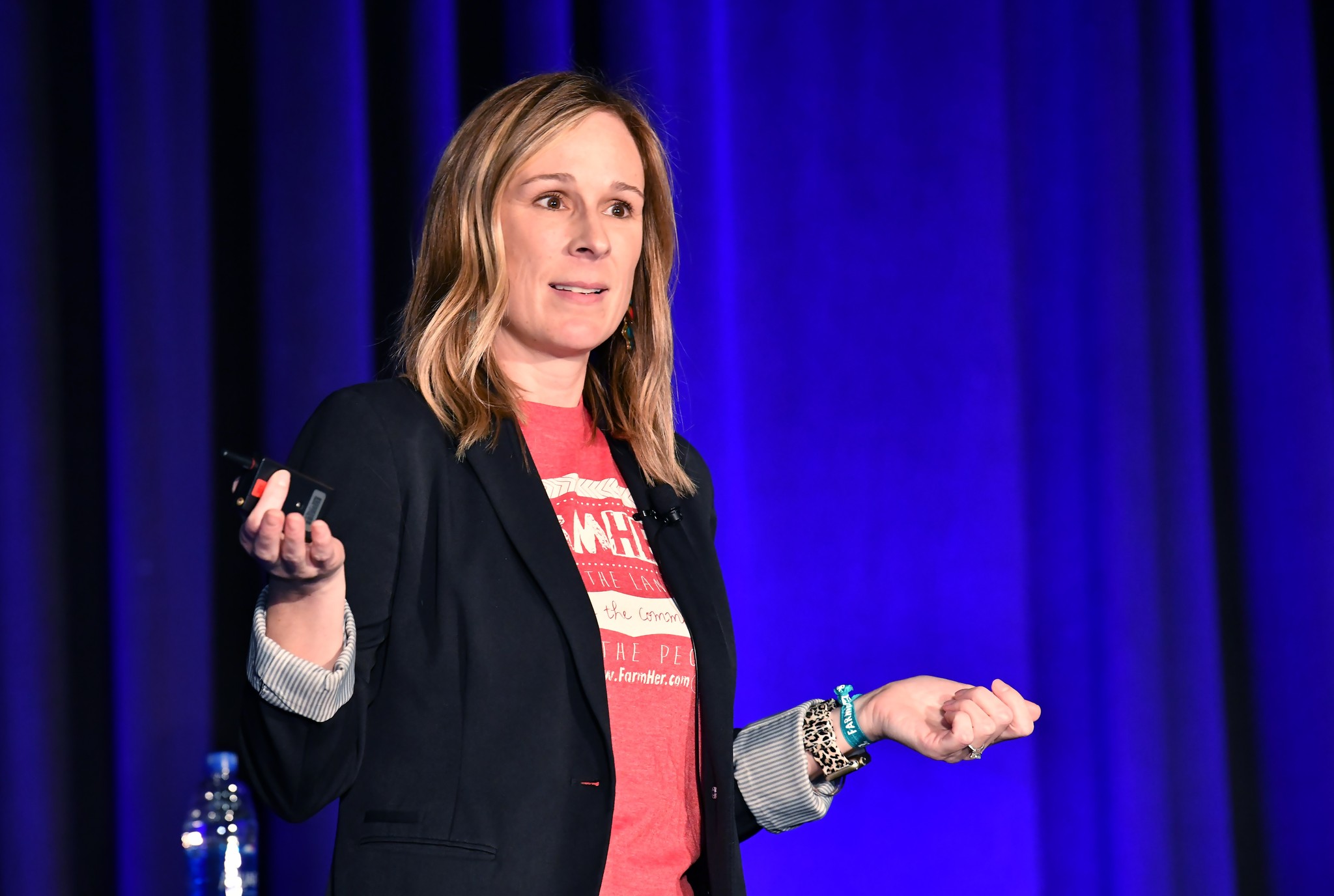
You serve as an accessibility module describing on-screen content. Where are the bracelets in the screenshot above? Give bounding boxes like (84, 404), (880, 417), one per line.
(834, 683), (874, 752)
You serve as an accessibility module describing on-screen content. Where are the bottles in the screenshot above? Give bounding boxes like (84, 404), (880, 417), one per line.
(180, 752), (260, 895)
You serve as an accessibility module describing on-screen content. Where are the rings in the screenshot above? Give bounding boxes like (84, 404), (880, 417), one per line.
(965, 744), (987, 759)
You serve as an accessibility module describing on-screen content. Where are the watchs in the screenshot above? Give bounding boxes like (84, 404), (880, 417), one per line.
(804, 697), (871, 782)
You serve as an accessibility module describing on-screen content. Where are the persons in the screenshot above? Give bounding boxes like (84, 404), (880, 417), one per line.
(238, 73), (1045, 896)
